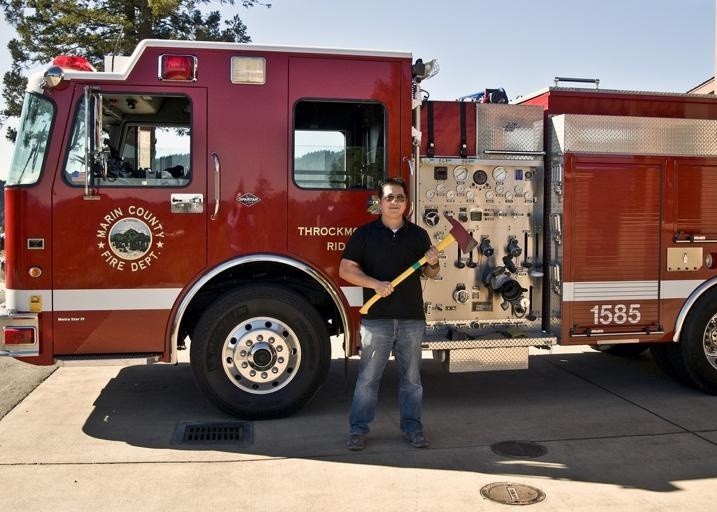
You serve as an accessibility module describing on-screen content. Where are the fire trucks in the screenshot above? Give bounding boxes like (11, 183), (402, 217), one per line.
(0, 34), (716, 425)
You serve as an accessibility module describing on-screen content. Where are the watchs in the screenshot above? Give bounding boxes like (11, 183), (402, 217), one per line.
(426, 260), (439, 269)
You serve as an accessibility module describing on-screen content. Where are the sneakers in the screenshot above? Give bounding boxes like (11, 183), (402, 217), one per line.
(345, 434), (369, 450)
(403, 431), (430, 448)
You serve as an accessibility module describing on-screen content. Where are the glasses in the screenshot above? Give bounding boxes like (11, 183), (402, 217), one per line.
(381, 194), (405, 202)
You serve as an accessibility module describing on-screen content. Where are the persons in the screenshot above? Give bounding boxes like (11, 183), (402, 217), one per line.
(338, 177), (440, 451)
